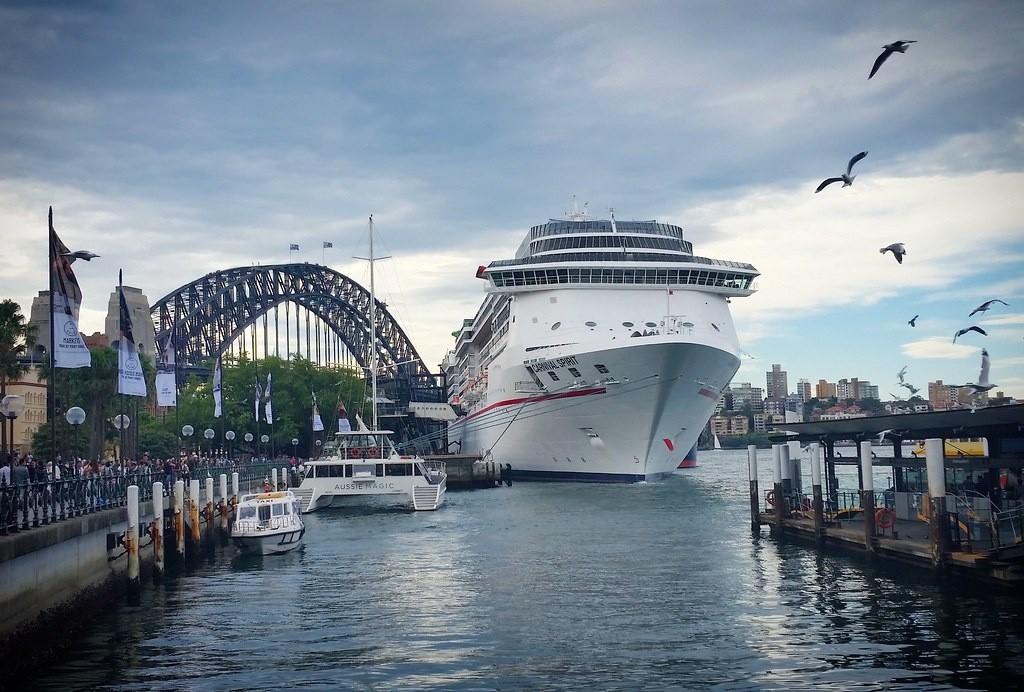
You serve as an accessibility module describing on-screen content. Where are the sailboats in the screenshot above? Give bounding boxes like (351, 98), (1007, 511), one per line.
(285, 214), (448, 516)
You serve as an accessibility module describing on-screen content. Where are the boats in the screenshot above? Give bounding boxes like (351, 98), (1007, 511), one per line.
(462, 371), (487, 402)
(231, 490), (306, 555)
(913, 438), (984, 455)
(451, 396), (460, 405)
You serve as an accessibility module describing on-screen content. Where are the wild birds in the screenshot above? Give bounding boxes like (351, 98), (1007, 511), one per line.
(875, 429), (902, 446)
(952, 326), (988, 345)
(815, 151), (868, 193)
(952, 348), (1000, 396)
(907, 314), (919, 328)
(967, 299), (1012, 316)
(879, 241), (907, 264)
(868, 40), (918, 80)
(889, 366), (921, 402)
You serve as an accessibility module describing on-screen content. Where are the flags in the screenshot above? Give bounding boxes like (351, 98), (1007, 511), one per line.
(668, 286), (673, 295)
(119, 288), (149, 396)
(212, 357), (221, 417)
(338, 396), (351, 432)
(265, 373), (272, 424)
(154, 327), (177, 407)
(52, 228), (91, 368)
(311, 391), (324, 432)
(255, 375), (262, 421)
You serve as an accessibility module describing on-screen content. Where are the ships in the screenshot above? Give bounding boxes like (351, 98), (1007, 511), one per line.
(442, 193), (761, 482)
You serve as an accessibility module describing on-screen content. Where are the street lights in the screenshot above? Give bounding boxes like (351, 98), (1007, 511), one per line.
(291, 437), (298, 459)
(245, 433), (253, 462)
(66, 407), (86, 507)
(262, 435), (269, 463)
(114, 415), (131, 498)
(316, 440), (322, 457)
(225, 431), (236, 464)
(0, 396), (25, 524)
(204, 429), (215, 467)
(182, 425), (195, 470)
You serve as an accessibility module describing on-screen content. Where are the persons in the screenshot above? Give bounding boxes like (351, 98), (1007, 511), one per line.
(264, 483), (271, 493)
(1017, 478), (1024, 500)
(0, 450), (239, 523)
(962, 476), (988, 497)
(243, 456), (303, 478)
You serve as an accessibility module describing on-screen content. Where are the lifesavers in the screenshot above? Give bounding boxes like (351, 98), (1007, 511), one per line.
(350, 448), (361, 458)
(368, 448), (378, 458)
(875, 509), (894, 528)
(766, 490), (774, 505)
